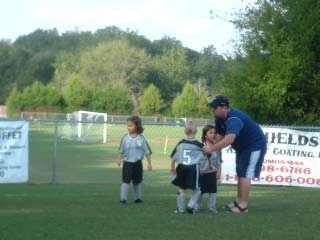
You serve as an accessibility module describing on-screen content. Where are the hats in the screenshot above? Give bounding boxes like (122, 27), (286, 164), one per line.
(208, 96), (229, 107)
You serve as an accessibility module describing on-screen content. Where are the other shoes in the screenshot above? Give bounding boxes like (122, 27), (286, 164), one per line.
(209, 207), (218, 214)
(174, 208), (184, 216)
(134, 199), (143, 203)
(187, 206), (196, 214)
(120, 200), (126, 206)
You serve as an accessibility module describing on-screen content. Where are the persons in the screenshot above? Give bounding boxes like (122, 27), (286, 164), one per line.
(200, 95), (267, 215)
(115, 116), (153, 205)
(169, 121), (215, 215)
(195, 125), (222, 214)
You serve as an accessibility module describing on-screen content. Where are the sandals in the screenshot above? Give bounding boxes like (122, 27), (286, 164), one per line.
(226, 204), (248, 214)
(222, 202), (237, 209)
